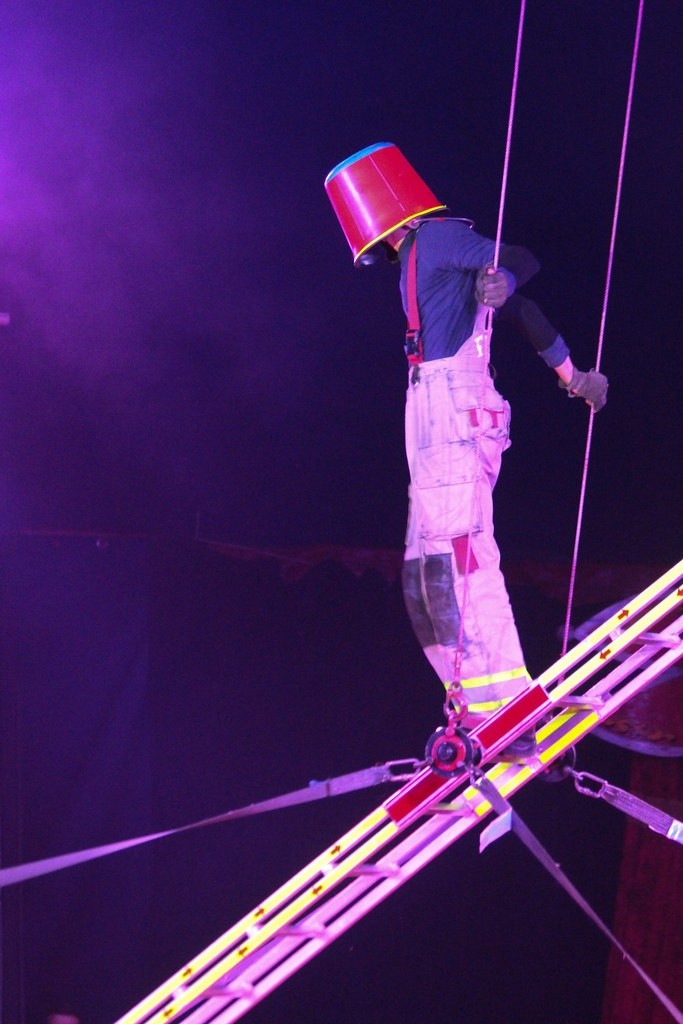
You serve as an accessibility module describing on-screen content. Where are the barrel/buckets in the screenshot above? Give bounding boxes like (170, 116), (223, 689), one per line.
(324, 141), (449, 270)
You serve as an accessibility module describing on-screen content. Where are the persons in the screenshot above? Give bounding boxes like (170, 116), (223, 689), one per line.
(324, 141), (610, 763)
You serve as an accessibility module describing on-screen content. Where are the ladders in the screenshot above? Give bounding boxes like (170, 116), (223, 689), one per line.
(111, 555), (683, 1024)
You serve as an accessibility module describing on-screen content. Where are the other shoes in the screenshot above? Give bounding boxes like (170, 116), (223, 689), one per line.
(486, 725), (535, 764)
